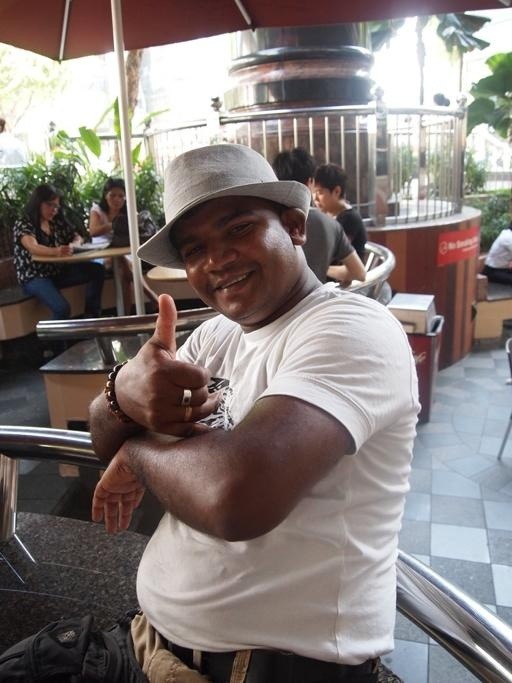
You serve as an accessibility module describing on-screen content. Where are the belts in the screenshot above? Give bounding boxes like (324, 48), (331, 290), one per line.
(157, 631), (379, 682)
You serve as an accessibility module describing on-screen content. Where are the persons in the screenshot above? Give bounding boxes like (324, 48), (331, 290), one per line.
(269, 147), (367, 289)
(12, 185), (103, 363)
(0, 118), (27, 168)
(89, 179), (159, 315)
(481, 223), (511, 286)
(87, 142), (421, 682)
(310, 165), (367, 263)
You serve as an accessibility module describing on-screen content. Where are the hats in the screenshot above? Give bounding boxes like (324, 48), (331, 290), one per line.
(132, 139), (318, 273)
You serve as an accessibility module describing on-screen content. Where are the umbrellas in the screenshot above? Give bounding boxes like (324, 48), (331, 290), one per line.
(1, 1), (512, 336)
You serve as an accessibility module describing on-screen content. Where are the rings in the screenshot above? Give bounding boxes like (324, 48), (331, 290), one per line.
(183, 405), (194, 424)
(181, 388), (192, 407)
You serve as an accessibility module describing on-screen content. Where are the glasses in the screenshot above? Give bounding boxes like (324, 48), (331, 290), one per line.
(45, 201), (60, 208)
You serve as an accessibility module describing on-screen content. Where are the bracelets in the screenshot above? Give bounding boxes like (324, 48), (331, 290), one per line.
(104, 361), (133, 423)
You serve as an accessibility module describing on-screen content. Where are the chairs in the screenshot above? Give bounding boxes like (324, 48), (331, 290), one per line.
(498, 337), (512, 459)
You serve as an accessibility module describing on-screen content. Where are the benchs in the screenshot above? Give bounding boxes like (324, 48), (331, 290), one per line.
(470, 248), (512, 356)
(0, 416), (511, 681)
(39, 241), (398, 481)
(1, 254), (157, 343)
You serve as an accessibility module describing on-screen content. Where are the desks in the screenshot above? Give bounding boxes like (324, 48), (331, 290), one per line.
(145, 264), (187, 281)
(28, 244), (168, 319)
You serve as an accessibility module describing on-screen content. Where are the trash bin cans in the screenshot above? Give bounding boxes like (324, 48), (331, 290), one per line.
(386, 293), (444, 423)
